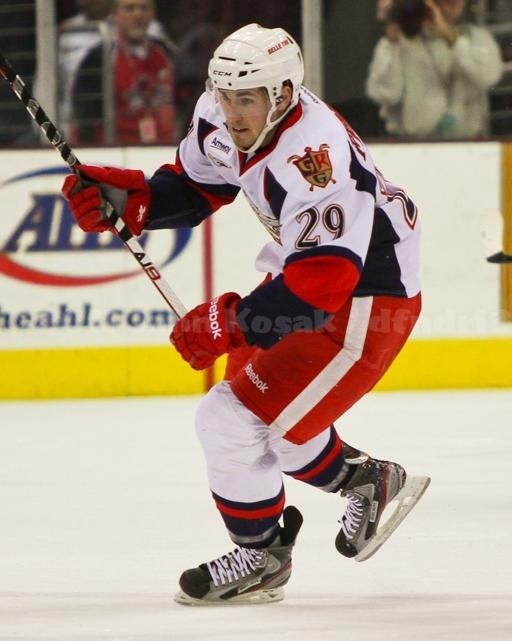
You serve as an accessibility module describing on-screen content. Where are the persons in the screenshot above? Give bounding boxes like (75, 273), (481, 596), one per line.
(368, 1), (502, 141)
(71, 1), (189, 145)
(60, 22), (422, 603)
(50, 2), (171, 145)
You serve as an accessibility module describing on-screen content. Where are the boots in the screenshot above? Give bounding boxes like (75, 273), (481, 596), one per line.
(174, 504), (304, 598)
(327, 441), (406, 558)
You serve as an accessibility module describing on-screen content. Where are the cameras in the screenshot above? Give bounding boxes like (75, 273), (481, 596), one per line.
(387, 1), (432, 40)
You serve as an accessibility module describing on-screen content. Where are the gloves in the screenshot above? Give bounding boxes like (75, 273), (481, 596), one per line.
(58, 157), (149, 243)
(165, 286), (235, 372)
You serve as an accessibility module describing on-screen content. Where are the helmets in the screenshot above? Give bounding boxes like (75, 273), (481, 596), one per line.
(203, 23), (303, 159)
